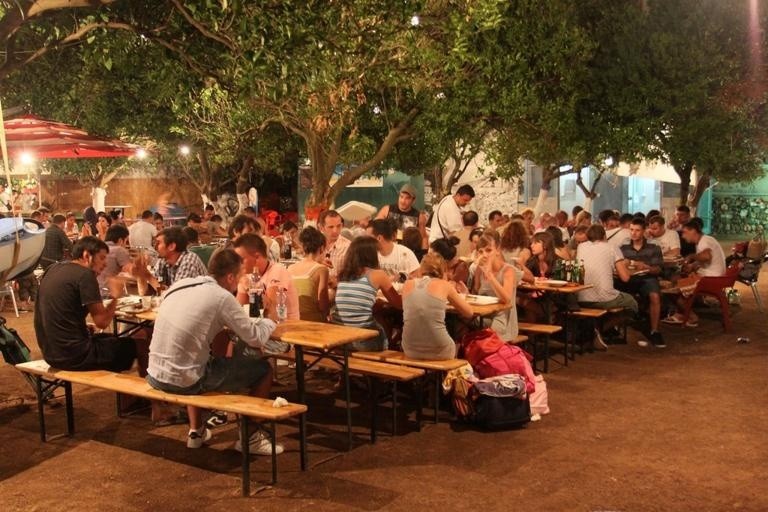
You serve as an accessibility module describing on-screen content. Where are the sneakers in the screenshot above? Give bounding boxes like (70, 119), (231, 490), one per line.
(591, 328), (609, 352)
(233, 429), (284, 457)
(206, 411), (229, 428)
(186, 426), (212, 450)
(646, 329), (667, 348)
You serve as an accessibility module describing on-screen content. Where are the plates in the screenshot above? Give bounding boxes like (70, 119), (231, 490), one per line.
(467, 294), (499, 306)
(544, 281), (567, 287)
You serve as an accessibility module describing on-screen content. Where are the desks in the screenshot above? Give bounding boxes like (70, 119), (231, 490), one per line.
(103, 205), (133, 218)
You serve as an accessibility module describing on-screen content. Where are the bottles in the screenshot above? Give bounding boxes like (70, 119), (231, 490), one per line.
(728, 289), (741, 305)
(247, 266), (264, 317)
(156, 277), (165, 295)
(553, 257), (585, 285)
(275, 286), (288, 325)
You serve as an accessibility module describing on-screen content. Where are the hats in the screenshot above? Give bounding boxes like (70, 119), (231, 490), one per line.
(401, 183), (416, 198)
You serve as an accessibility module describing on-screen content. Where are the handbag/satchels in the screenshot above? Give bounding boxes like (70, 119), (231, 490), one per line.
(211, 329), (267, 361)
(724, 232), (766, 283)
(441, 326), (551, 431)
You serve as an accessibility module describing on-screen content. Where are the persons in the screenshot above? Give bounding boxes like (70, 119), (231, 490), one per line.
(26, 184), (726, 364)
(35, 236), (189, 426)
(0, 177), (11, 212)
(146, 250), (284, 455)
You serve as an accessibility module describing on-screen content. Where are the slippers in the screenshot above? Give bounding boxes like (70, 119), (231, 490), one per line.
(663, 315), (684, 324)
(680, 320), (699, 328)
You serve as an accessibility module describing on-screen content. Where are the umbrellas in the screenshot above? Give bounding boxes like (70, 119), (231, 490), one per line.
(1, 113), (153, 207)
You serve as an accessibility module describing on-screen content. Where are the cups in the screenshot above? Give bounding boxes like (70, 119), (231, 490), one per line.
(142, 296), (152, 312)
